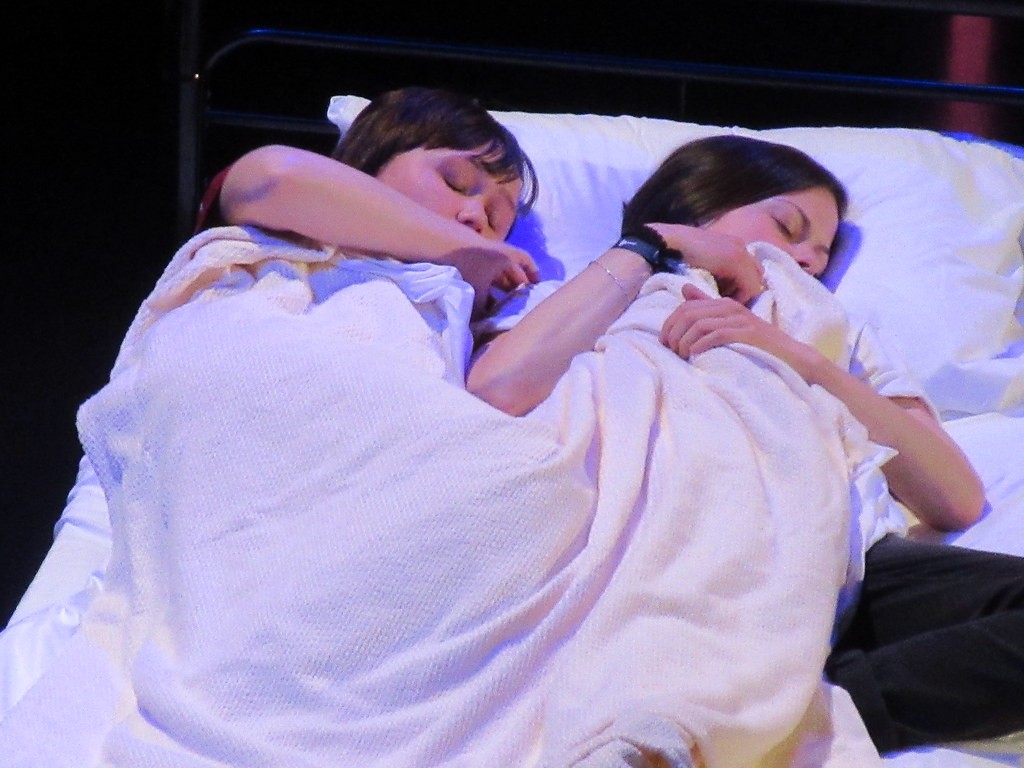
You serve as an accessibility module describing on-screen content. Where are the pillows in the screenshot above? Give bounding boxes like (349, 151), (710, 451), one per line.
(325, 90), (1024, 412)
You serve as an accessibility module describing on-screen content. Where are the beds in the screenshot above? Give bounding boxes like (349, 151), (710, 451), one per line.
(0, 31), (1024, 768)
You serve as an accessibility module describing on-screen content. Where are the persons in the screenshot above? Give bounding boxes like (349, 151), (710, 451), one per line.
(191, 91), (540, 322)
(467, 134), (986, 530)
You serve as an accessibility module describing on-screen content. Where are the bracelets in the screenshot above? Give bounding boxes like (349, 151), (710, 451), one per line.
(612, 236), (681, 273)
(590, 260), (630, 306)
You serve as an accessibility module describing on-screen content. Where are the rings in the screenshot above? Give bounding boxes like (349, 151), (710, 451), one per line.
(760, 285), (765, 291)
